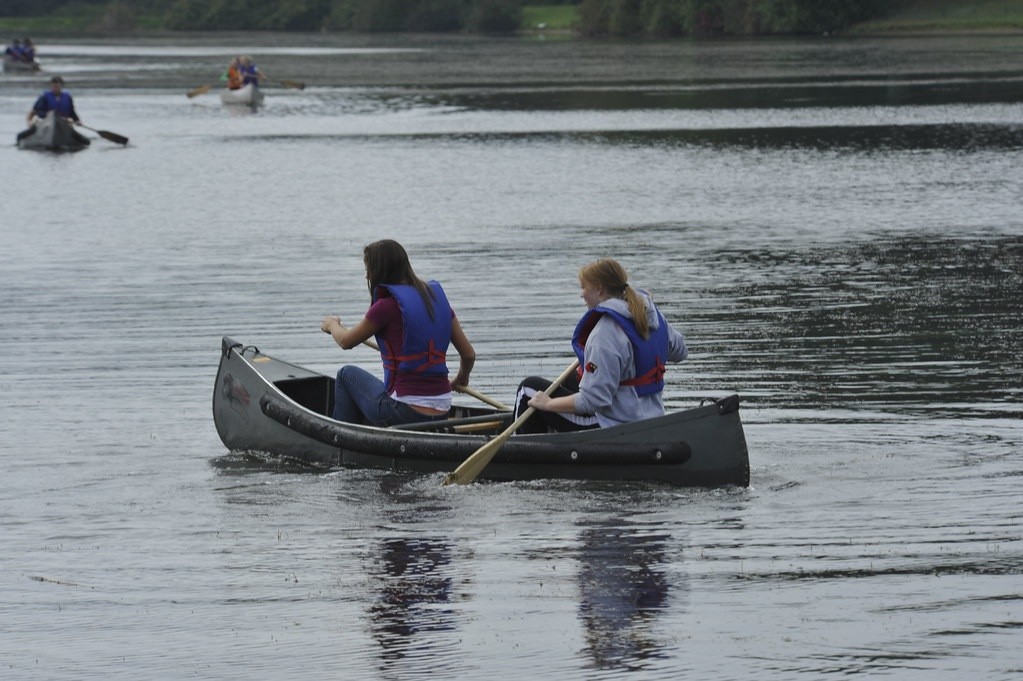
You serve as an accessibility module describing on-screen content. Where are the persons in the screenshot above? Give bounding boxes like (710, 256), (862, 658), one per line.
(27, 76), (81, 127)
(6, 38), (34, 63)
(220, 55), (270, 91)
(512, 257), (688, 435)
(321, 239), (476, 428)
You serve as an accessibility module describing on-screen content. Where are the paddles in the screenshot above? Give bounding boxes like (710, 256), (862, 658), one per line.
(248, 74), (307, 91)
(441, 357), (582, 488)
(323, 316), (514, 411)
(68, 120), (129, 145)
(186, 80), (224, 99)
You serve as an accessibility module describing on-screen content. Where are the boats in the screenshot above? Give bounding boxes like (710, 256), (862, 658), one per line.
(221, 81), (265, 106)
(18, 110), (92, 152)
(212, 335), (750, 487)
(4, 58), (41, 74)
